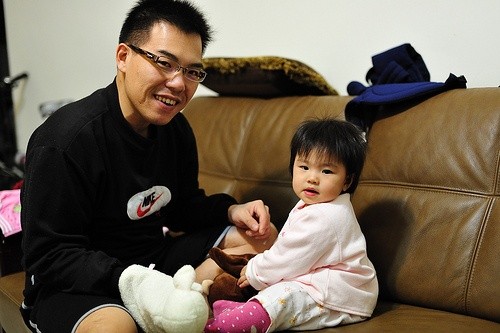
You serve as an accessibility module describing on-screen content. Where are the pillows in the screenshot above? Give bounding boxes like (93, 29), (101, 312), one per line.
(198, 56), (339, 96)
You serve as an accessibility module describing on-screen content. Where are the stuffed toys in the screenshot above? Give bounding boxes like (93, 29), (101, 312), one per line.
(203, 246), (261, 316)
(116, 261), (213, 333)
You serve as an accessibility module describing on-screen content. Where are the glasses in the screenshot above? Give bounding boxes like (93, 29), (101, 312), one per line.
(126, 43), (207, 84)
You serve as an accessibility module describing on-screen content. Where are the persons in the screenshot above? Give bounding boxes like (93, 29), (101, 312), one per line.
(20, 1), (278, 333)
(204, 111), (379, 333)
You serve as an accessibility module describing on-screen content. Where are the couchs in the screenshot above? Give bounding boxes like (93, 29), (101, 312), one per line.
(0, 87), (500, 333)
